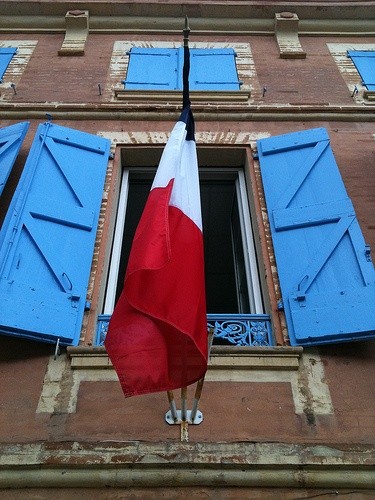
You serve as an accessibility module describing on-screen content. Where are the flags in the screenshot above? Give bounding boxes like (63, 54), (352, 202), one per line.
(103, 105), (209, 399)
